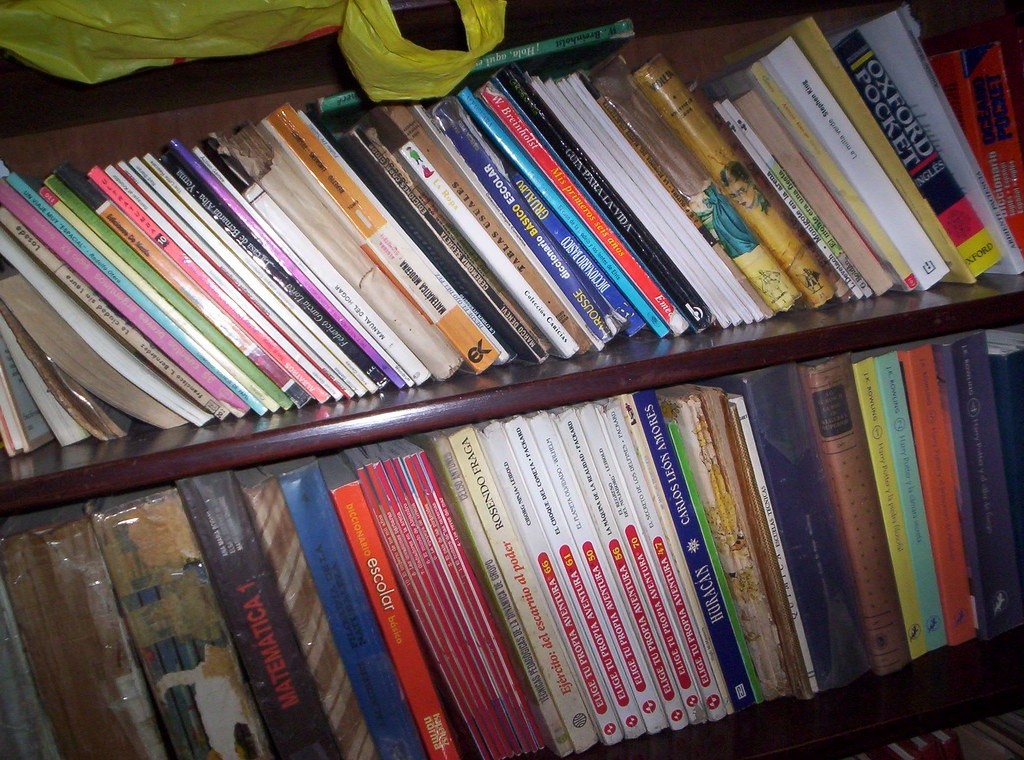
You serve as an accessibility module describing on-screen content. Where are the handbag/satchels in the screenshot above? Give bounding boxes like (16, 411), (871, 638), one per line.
(0, 0), (506, 102)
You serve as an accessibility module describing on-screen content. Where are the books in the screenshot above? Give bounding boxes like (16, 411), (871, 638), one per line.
(0, 1), (1024, 456)
(0, 331), (1024, 760)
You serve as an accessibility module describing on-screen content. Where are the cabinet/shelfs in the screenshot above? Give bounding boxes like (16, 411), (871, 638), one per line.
(0, 0), (1024, 760)
